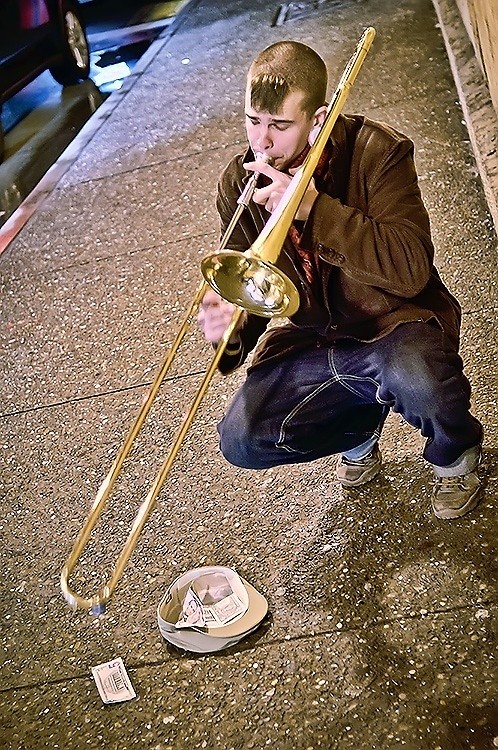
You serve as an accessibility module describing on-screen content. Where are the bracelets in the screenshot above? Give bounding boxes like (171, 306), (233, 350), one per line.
(225, 336), (242, 355)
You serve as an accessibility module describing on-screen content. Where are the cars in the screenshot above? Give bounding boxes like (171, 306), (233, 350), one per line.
(0, 0), (91, 110)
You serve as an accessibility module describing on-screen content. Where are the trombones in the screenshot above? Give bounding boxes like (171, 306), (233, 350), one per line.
(56, 26), (380, 615)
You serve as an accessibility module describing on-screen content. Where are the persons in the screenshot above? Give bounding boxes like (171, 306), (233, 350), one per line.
(196, 41), (484, 519)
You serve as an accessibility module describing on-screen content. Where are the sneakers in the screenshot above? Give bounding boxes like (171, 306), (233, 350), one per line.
(336, 443), (382, 489)
(431, 467), (481, 519)
